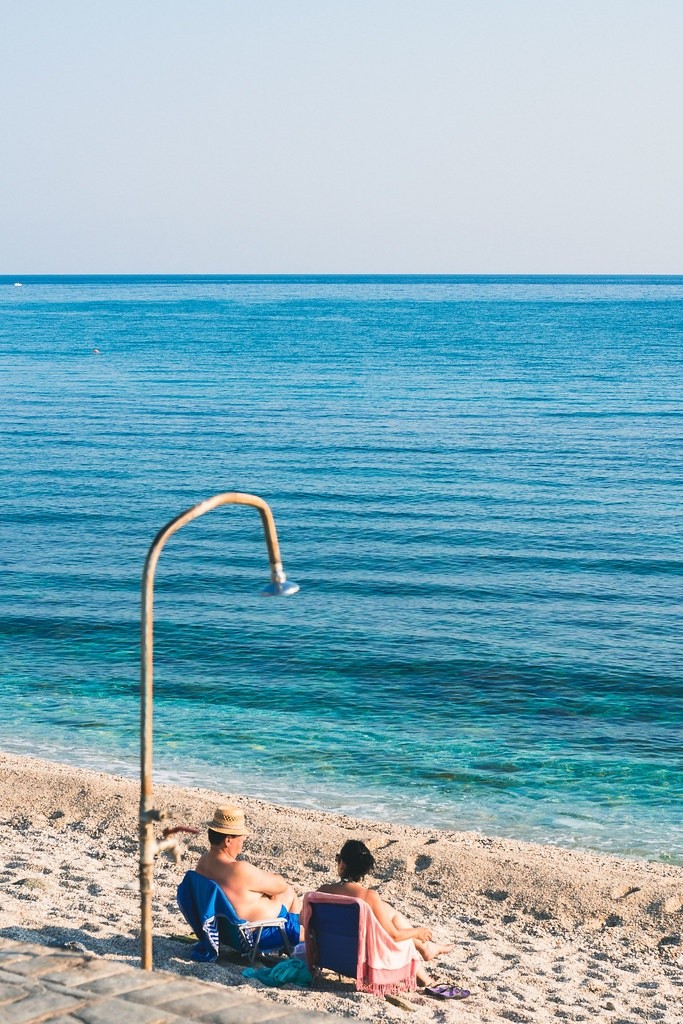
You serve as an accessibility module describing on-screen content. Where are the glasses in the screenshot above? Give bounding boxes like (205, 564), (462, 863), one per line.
(335, 853), (340, 862)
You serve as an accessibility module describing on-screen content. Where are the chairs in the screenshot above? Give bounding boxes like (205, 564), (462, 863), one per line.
(175, 871), (294, 967)
(298, 892), (419, 995)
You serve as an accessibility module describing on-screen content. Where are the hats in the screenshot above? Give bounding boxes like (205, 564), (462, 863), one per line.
(206, 805), (253, 836)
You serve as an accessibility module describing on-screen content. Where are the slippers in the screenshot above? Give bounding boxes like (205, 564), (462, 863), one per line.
(438, 985), (470, 999)
(425, 982), (453, 994)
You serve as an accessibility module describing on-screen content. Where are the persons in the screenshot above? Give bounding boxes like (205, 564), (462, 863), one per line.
(194, 806), (305, 953)
(298, 840), (452, 990)
